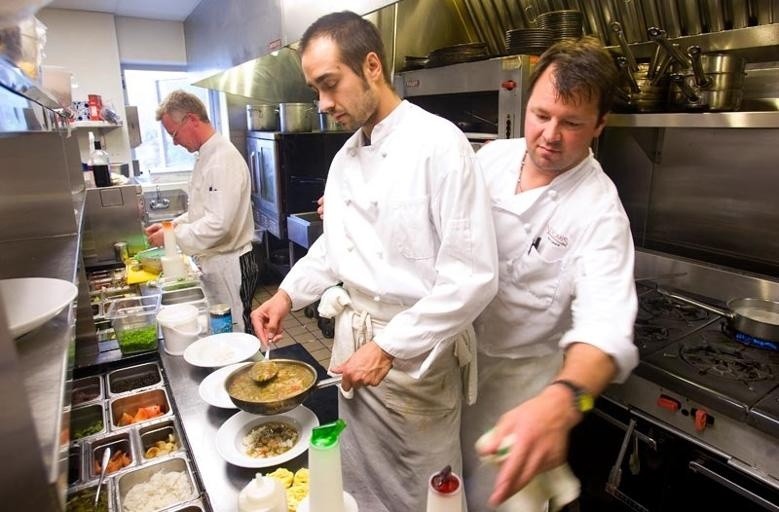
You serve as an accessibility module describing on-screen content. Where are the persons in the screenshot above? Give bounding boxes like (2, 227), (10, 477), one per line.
(317, 34), (642, 512)
(143, 89), (260, 332)
(250, 12), (499, 512)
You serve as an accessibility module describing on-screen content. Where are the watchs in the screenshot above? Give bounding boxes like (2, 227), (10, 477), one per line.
(549, 380), (594, 419)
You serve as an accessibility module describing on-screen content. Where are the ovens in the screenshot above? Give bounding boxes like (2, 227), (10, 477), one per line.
(381, 54), (538, 157)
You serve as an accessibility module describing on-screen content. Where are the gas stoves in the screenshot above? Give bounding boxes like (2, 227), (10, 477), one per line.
(595, 277), (779, 478)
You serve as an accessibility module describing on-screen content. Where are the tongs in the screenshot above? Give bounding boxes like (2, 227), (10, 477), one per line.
(604, 418), (642, 495)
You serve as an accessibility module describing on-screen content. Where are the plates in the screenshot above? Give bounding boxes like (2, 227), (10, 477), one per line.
(0, 277), (79, 342)
(214, 402), (321, 469)
(198, 361), (255, 411)
(504, 8), (583, 55)
(183, 333), (262, 368)
(404, 40), (489, 70)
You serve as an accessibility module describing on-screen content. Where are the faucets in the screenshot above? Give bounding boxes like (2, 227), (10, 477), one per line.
(155, 184), (161, 202)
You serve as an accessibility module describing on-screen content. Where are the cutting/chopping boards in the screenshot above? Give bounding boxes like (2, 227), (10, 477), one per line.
(124, 253), (192, 284)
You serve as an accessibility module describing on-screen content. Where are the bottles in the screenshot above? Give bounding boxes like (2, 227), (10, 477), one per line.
(425, 464), (463, 512)
(88, 94), (104, 120)
(209, 303), (233, 334)
(294, 415), (360, 512)
(90, 140), (111, 188)
(160, 221), (185, 278)
(236, 470), (289, 512)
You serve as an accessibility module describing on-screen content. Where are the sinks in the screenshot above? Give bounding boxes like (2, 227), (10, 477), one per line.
(286, 211), (324, 250)
(141, 181), (190, 193)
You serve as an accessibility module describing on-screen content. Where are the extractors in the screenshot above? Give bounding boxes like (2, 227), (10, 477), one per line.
(455, 1), (778, 65)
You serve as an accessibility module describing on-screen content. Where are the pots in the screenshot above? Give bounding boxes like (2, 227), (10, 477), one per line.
(654, 286), (779, 342)
(226, 357), (345, 414)
(599, 21), (750, 111)
(277, 102), (316, 132)
(245, 102), (277, 132)
(314, 99), (353, 132)
